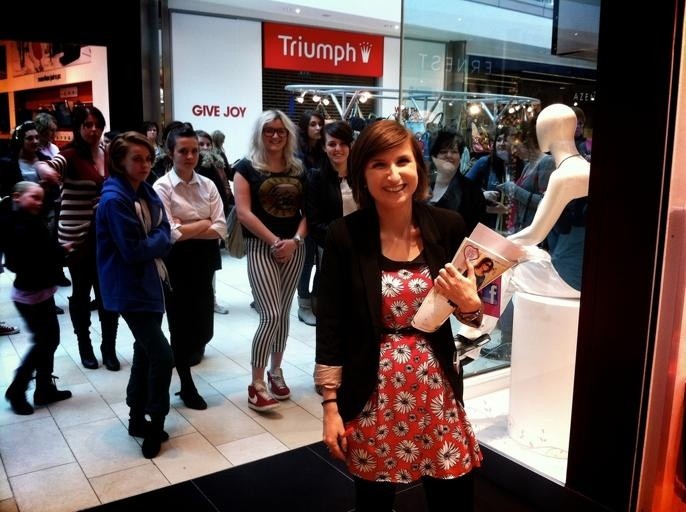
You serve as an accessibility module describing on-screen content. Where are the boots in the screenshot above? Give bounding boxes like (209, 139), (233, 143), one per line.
(298, 296), (316, 326)
(68, 296), (120, 371)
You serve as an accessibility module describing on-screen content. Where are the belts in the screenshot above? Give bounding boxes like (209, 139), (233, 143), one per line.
(385, 326), (417, 335)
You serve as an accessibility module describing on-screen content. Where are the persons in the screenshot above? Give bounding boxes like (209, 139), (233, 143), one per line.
(308, 121), (359, 267)
(430, 257), (493, 327)
(480, 301), (512, 361)
(453, 103), (591, 366)
(95, 131), (174, 458)
(140, 121), (164, 157)
(312, 120), (483, 512)
(1, 181), (77, 415)
(424, 106), (556, 359)
(295, 111), (325, 326)
(152, 121), (239, 410)
(232, 109), (311, 412)
(152, 127), (228, 410)
(33, 105), (121, 370)
(1, 105), (121, 370)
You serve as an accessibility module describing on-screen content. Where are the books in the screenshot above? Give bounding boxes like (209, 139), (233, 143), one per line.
(411, 237), (518, 332)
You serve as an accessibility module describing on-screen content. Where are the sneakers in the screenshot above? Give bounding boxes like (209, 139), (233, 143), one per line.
(6, 384), (72, 415)
(214, 304), (228, 314)
(248, 368), (290, 411)
(481, 344), (511, 360)
(0, 325), (20, 336)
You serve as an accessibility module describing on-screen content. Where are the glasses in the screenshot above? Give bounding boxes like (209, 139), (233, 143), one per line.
(264, 128), (288, 138)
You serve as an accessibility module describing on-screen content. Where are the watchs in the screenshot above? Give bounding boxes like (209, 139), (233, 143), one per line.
(458, 307), (482, 324)
(293, 235), (304, 245)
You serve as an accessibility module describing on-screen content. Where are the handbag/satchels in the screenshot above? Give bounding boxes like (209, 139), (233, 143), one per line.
(225, 207), (246, 257)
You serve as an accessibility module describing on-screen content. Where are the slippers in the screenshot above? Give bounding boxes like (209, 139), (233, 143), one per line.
(454, 334), (491, 355)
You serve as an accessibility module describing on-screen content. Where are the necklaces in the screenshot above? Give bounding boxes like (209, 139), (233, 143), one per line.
(559, 154), (582, 169)
(339, 176), (347, 183)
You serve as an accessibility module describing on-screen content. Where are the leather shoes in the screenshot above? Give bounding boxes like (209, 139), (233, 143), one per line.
(129, 420), (169, 459)
(55, 276), (70, 313)
(180, 390), (207, 409)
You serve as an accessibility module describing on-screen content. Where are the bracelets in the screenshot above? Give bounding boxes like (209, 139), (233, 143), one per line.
(271, 237), (281, 248)
(321, 399), (338, 406)
